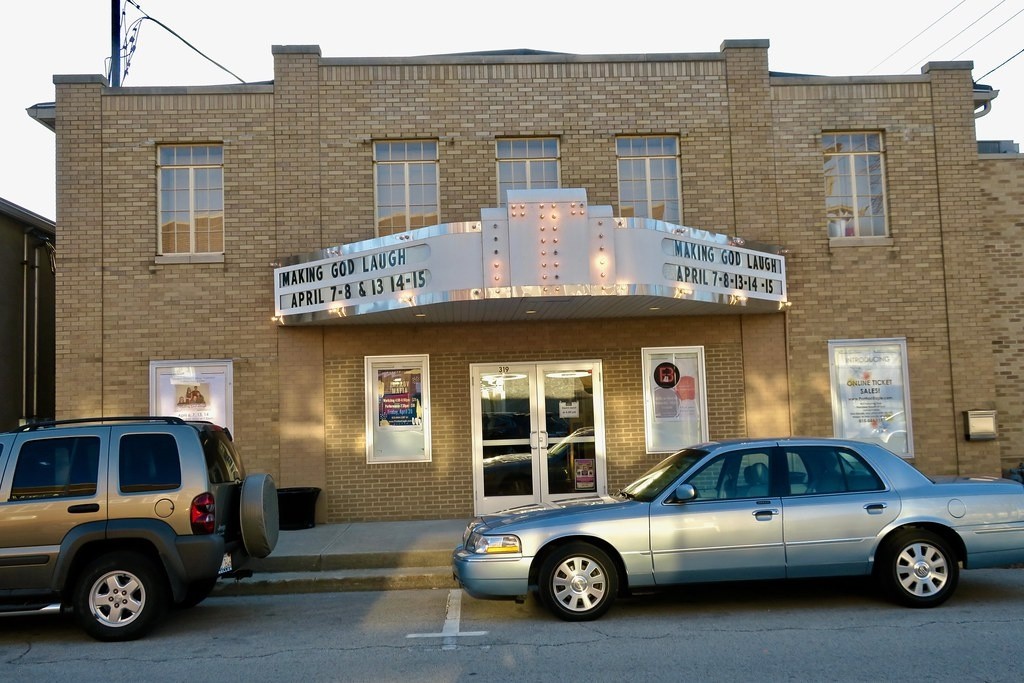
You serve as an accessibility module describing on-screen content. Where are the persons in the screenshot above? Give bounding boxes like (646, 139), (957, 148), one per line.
(177, 384), (206, 404)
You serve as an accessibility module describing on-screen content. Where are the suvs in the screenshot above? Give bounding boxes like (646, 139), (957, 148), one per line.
(0, 414), (280, 642)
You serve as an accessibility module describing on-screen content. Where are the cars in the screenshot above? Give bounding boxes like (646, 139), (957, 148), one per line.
(450, 435), (1024, 623)
(483, 426), (595, 497)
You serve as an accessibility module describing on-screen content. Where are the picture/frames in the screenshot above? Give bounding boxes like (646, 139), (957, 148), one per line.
(363, 353), (433, 464)
(640, 345), (709, 454)
(149, 359), (235, 443)
(825, 337), (915, 460)
